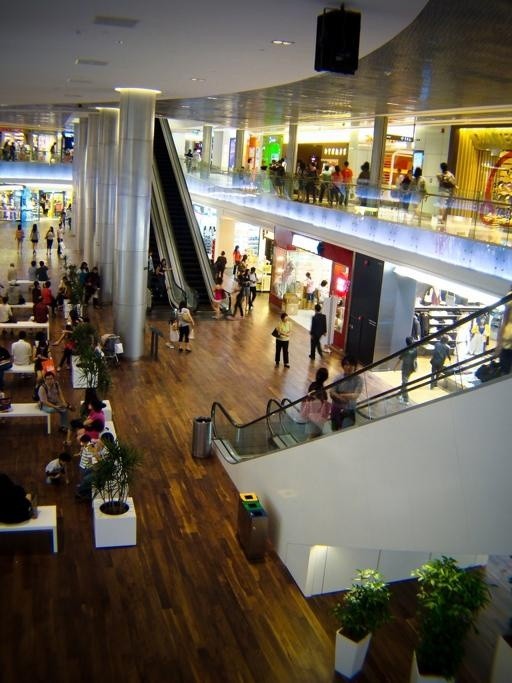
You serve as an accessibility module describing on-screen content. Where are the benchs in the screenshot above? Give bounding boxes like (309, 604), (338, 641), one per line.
(89, 420), (129, 507)
(0, 504), (58, 554)
(0, 280), (50, 435)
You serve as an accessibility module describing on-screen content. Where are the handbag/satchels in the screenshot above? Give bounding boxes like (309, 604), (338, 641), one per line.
(182, 313), (192, 323)
(272, 328), (280, 338)
(300, 396), (332, 425)
(475, 353), (504, 381)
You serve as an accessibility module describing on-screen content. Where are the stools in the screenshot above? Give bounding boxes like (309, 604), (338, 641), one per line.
(81, 400), (114, 420)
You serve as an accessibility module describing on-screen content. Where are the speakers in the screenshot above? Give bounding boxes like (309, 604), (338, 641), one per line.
(314, 9), (362, 76)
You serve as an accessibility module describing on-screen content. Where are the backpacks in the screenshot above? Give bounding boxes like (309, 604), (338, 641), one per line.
(32, 382), (48, 401)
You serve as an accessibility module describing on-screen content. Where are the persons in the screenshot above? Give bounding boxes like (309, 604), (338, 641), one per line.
(2, 261), (84, 400)
(16, 223), (64, 254)
(51, 143), (57, 154)
(396, 163), (455, 223)
(240, 157), (286, 197)
(212, 245), (258, 319)
(4, 140), (15, 162)
(169, 301), (194, 352)
(148, 251), (171, 297)
(59, 204), (71, 229)
(185, 148), (200, 173)
(306, 272), (328, 310)
(77, 261), (100, 310)
(394, 337), (418, 405)
(38, 372), (117, 501)
(493, 292), (512, 375)
(309, 304), (327, 360)
(274, 313), (292, 369)
(430, 337), (451, 389)
(305, 355), (365, 441)
(297, 158), (370, 208)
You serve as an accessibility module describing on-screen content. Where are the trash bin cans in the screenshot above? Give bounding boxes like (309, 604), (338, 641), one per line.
(237, 492), (269, 560)
(191, 416), (213, 458)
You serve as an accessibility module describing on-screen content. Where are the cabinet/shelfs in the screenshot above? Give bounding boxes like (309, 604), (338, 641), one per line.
(414, 307), (484, 343)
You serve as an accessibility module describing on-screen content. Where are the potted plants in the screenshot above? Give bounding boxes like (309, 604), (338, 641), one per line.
(325, 566), (395, 679)
(406, 553), (500, 681)
(81, 433), (147, 550)
(63, 264), (114, 400)
